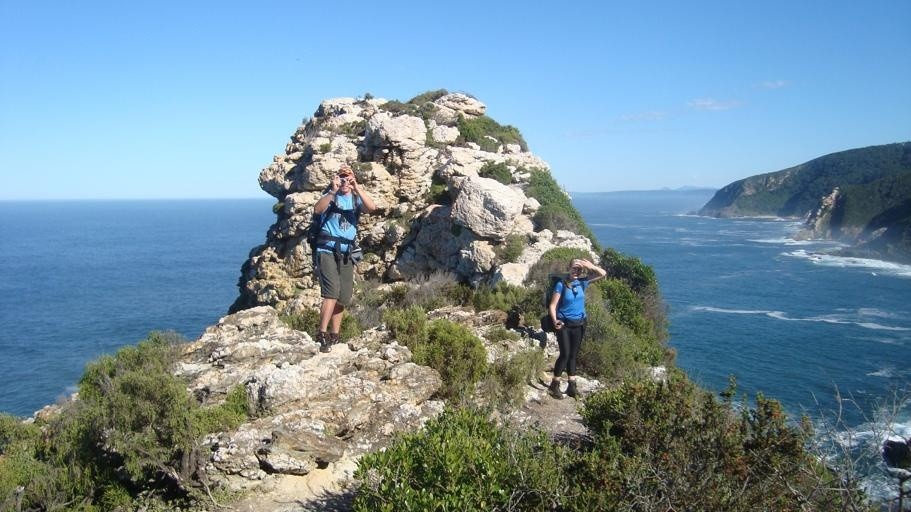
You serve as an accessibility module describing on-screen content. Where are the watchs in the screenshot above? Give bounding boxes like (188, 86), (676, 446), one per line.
(328, 189), (337, 196)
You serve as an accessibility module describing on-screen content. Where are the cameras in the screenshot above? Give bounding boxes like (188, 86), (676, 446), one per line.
(339, 178), (348, 187)
(558, 321), (565, 326)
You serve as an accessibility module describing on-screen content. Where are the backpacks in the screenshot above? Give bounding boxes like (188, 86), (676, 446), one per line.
(540, 274), (585, 333)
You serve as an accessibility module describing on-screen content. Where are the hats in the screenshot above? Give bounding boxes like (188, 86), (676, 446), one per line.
(338, 168), (354, 176)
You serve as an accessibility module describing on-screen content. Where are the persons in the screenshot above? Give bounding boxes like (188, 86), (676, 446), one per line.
(312, 167), (375, 353)
(547, 258), (606, 399)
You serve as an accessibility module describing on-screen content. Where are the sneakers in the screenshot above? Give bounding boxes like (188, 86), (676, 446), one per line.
(566, 380), (580, 398)
(316, 336), (331, 352)
(330, 333), (339, 345)
(549, 381), (562, 398)
